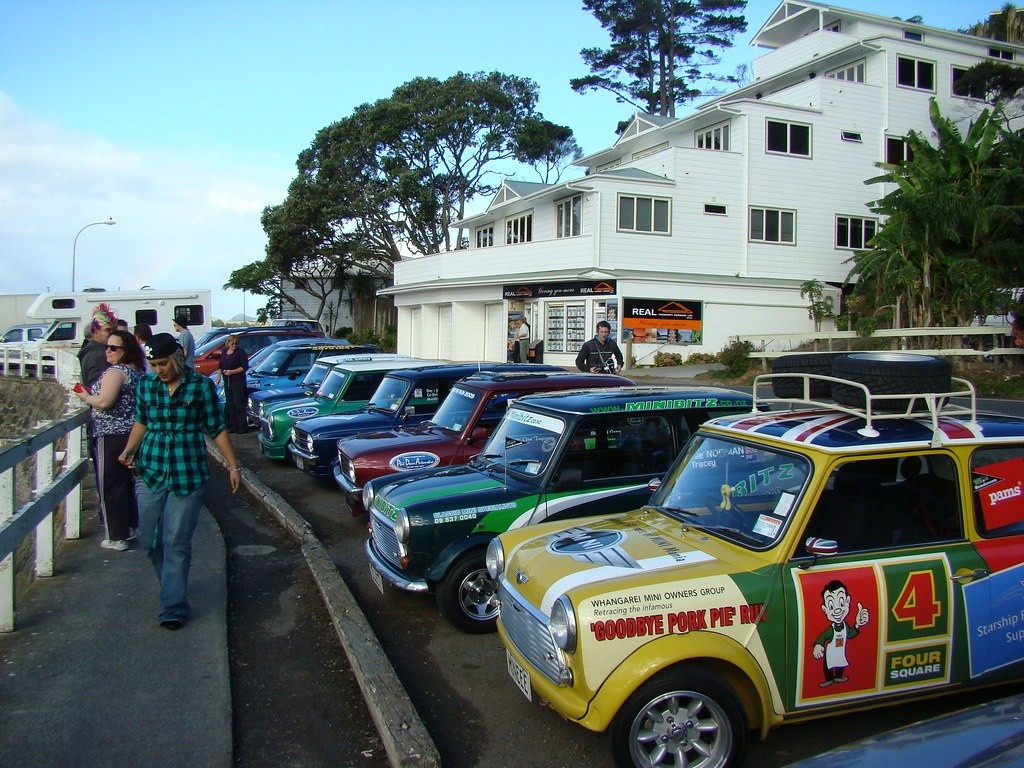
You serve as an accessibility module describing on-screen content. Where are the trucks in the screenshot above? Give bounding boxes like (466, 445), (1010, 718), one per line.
(25, 287), (211, 374)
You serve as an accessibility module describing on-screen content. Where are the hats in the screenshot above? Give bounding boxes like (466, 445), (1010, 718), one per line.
(172, 316), (189, 330)
(144, 332), (179, 359)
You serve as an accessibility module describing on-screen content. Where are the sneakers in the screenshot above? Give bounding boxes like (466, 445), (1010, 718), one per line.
(101, 538), (130, 551)
(126, 527), (136, 541)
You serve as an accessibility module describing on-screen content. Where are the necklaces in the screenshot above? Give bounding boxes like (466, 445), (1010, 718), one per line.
(167, 377), (178, 392)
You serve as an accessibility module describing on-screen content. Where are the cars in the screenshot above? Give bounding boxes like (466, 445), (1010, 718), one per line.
(333, 371), (638, 517)
(246, 354), (420, 428)
(207, 337), (351, 388)
(961, 287), (1024, 351)
(257, 360), (453, 461)
(194, 328), (251, 348)
(0, 323), (73, 367)
(215, 343), (377, 424)
(485, 373), (1023, 768)
(269, 319), (329, 343)
(286, 362), (567, 478)
(362, 386), (772, 633)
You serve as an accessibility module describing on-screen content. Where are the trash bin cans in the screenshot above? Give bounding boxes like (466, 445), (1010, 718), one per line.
(528, 339), (543, 364)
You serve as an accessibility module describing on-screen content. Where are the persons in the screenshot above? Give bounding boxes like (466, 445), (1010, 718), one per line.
(215, 335), (248, 434)
(512, 317), (530, 363)
(575, 321), (624, 375)
(118, 333), (241, 631)
(76, 303), (195, 526)
(71, 329), (148, 550)
(1004, 294), (1024, 382)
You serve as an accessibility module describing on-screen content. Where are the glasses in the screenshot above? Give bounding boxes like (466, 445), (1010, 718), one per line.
(104, 344), (126, 351)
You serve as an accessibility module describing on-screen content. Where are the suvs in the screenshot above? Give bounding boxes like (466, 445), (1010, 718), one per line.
(193, 326), (325, 376)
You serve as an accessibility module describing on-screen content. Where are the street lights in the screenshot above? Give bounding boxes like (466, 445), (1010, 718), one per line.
(72, 222), (116, 292)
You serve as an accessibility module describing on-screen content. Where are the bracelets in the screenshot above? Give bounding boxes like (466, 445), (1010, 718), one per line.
(227, 465), (240, 472)
(84, 395), (91, 407)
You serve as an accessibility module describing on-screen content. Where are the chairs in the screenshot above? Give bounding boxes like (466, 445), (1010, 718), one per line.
(821, 463), (917, 548)
